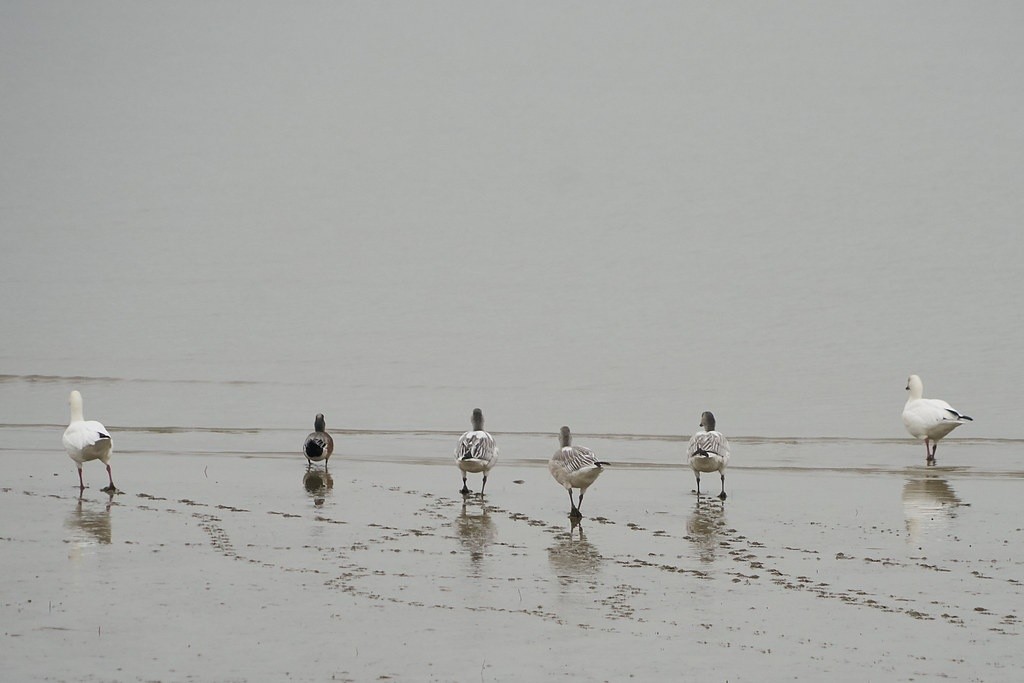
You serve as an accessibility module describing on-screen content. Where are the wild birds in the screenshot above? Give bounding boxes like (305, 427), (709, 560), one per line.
(548, 426), (612, 518)
(686, 411), (732, 499)
(302, 413), (335, 469)
(901, 374), (974, 462)
(62, 390), (121, 493)
(453, 408), (499, 496)
